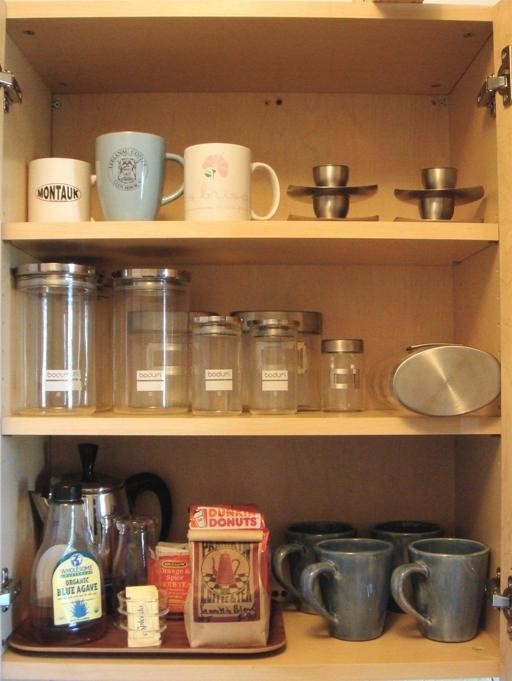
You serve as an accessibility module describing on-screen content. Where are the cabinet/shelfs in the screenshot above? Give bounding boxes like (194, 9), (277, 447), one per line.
(1, 0), (512, 681)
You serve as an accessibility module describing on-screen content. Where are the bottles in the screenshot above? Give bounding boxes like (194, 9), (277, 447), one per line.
(111, 514), (155, 628)
(10, 263), (98, 417)
(191, 315), (244, 416)
(246, 318), (298, 414)
(28, 483), (107, 645)
(321, 339), (364, 413)
(231, 310), (323, 411)
(112, 267), (192, 414)
(126, 310), (218, 411)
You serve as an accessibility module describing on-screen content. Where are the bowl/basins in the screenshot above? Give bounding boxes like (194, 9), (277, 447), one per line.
(313, 164), (349, 185)
(314, 193), (351, 218)
(418, 197), (452, 219)
(422, 166), (459, 188)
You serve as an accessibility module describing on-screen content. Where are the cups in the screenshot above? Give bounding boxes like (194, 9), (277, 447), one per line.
(391, 538), (490, 642)
(185, 143), (279, 219)
(376, 520), (440, 612)
(93, 132), (185, 222)
(28, 159), (97, 222)
(299, 539), (392, 641)
(273, 520), (356, 614)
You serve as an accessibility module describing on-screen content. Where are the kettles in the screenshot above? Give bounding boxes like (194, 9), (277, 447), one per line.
(30, 444), (173, 615)
